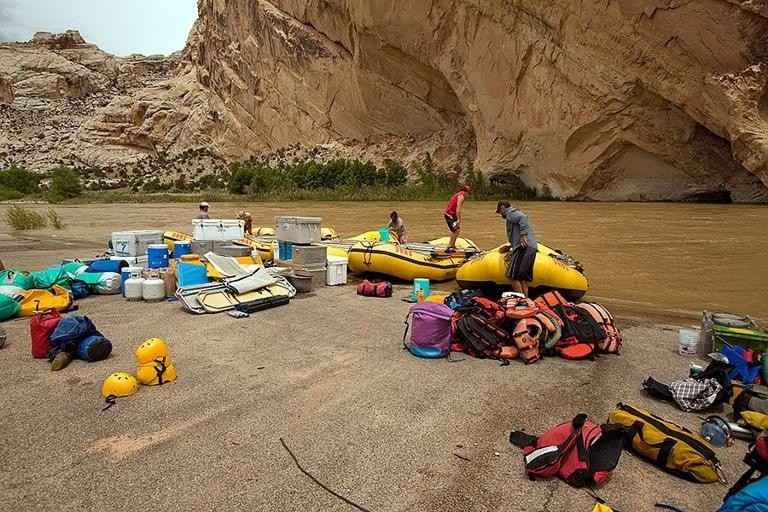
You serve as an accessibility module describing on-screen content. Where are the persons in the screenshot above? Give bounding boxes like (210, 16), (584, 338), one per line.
(441, 183), (473, 252)
(104, 240), (115, 256)
(493, 198), (538, 298)
(386, 209), (408, 243)
(5, 271), (15, 283)
(191, 201), (212, 219)
(235, 211), (254, 236)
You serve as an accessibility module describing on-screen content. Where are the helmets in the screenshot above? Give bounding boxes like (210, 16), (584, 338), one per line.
(102, 372), (138, 397)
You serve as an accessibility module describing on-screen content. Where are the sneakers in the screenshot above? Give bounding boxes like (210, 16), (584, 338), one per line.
(444, 246), (456, 253)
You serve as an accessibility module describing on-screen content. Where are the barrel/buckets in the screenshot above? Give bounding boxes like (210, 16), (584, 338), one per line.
(380, 229), (389, 240)
(148, 244), (168, 268)
(678, 330), (700, 356)
(410, 278), (430, 301)
(700, 418), (731, 446)
(175, 241), (192, 256)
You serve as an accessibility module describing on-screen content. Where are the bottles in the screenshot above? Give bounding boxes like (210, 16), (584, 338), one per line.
(416, 287), (424, 304)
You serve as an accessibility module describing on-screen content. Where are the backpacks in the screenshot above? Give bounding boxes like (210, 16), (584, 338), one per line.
(669, 378), (722, 412)
(456, 313), (509, 359)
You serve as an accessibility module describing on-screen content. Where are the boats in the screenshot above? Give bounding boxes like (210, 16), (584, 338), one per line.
(457, 239), (587, 299)
(270, 230), (398, 257)
(232, 228), (340, 252)
(154, 225), (275, 260)
(347, 234), (479, 282)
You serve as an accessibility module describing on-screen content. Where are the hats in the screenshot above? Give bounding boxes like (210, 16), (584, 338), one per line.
(199, 201), (210, 207)
(463, 185), (470, 194)
(496, 200), (511, 213)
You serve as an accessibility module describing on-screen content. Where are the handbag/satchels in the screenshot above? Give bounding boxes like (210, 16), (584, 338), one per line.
(49, 314), (104, 347)
(356, 278), (393, 298)
(606, 402), (728, 485)
(403, 300), (453, 359)
(135, 338), (177, 386)
(30, 306), (63, 358)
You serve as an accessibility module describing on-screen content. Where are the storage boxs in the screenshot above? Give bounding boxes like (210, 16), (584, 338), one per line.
(110, 229), (166, 267)
(190, 218), (246, 256)
(273, 215), (349, 289)
